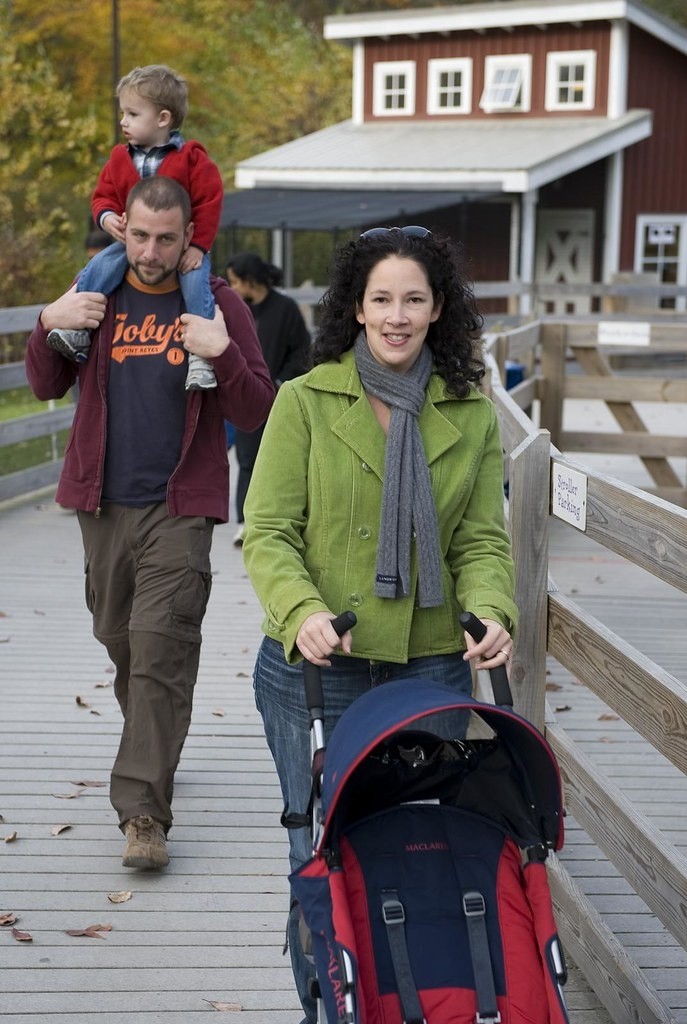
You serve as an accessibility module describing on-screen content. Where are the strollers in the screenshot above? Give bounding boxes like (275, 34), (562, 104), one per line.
(281, 612), (569, 1024)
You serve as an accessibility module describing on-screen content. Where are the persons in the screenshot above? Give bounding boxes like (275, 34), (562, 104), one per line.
(223, 254), (312, 547)
(24, 175), (276, 874)
(241, 227), (512, 1024)
(46, 65), (226, 393)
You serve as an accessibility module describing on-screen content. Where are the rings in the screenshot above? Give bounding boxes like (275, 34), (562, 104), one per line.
(498, 650), (509, 661)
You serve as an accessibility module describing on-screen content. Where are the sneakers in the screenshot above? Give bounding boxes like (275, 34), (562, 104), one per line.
(121, 813), (170, 868)
(46, 328), (91, 363)
(185, 353), (218, 390)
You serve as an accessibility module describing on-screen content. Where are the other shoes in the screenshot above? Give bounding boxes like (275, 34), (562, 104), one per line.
(233, 522), (244, 546)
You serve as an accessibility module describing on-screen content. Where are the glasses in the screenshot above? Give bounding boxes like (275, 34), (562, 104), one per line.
(359, 225), (434, 242)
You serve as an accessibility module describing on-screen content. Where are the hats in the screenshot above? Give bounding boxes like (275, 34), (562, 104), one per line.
(85, 229), (113, 248)
